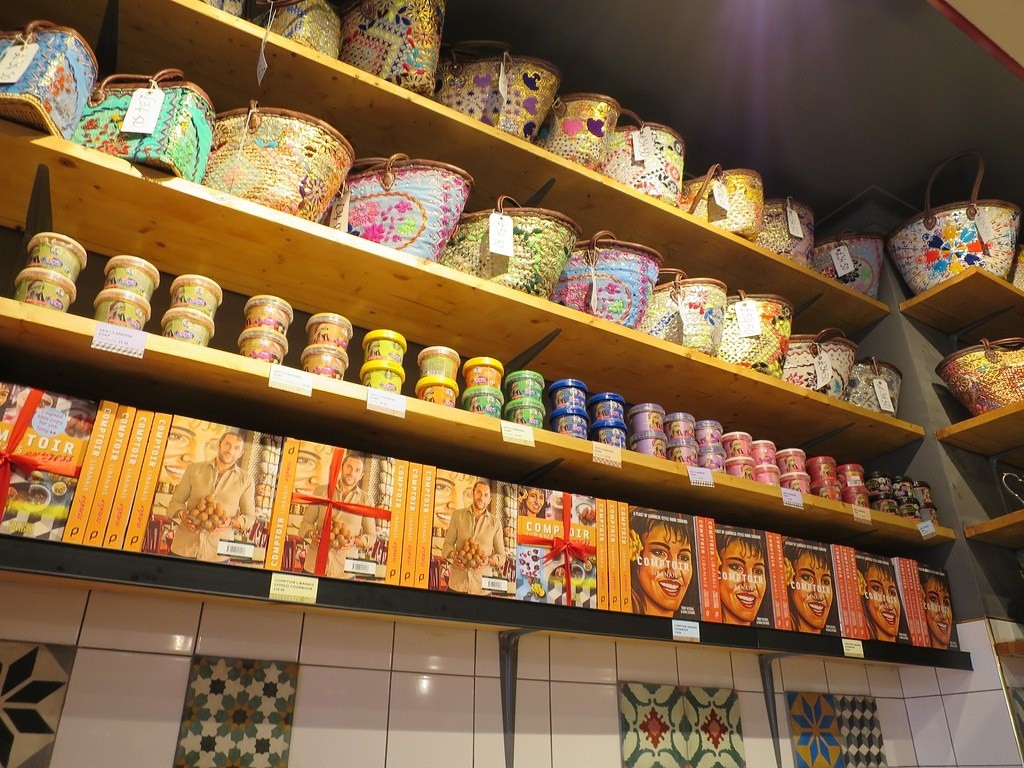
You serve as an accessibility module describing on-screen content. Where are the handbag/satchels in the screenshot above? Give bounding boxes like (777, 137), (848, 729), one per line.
(438, 194), (582, 299)
(638, 265), (729, 357)
(203, 99), (354, 221)
(0, 18), (98, 140)
(683, 163), (764, 240)
(935, 335), (1024, 417)
(849, 354), (905, 419)
(716, 288), (794, 376)
(755, 196), (815, 270)
(814, 227), (884, 299)
(884, 153), (1024, 296)
(201, 0), (685, 210)
(319, 154), (474, 262)
(547, 228), (663, 332)
(780, 327), (859, 399)
(70, 67), (216, 185)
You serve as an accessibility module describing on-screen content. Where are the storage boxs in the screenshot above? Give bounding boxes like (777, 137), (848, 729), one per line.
(0, 382), (960, 654)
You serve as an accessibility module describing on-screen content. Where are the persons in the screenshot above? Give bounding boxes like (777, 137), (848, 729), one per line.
(442, 481), (505, 595)
(300, 454), (376, 580)
(167, 433), (255, 561)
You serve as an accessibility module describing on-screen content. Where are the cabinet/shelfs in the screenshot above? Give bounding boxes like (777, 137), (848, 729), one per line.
(900, 265), (1023, 550)
(0, 0), (975, 674)
